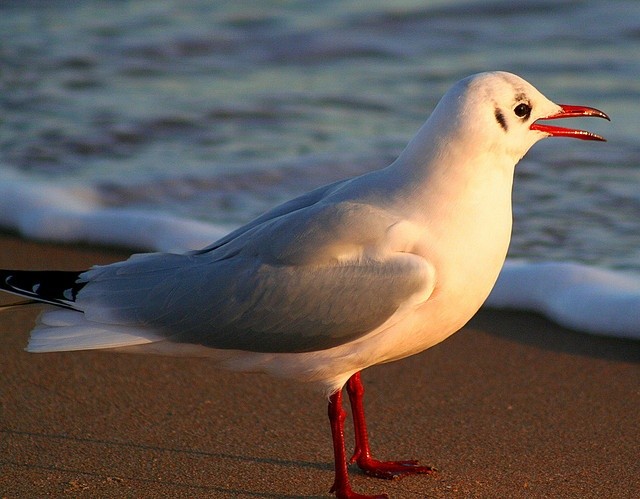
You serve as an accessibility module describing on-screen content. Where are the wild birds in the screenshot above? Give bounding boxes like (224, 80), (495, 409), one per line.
(0, 68), (614, 499)
